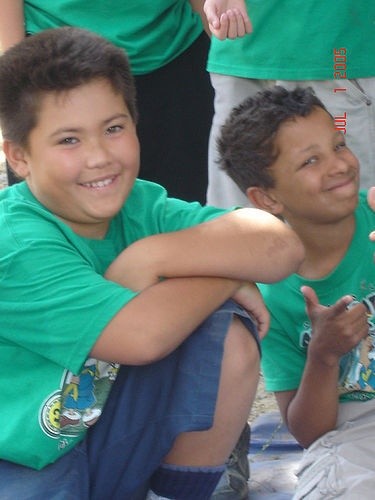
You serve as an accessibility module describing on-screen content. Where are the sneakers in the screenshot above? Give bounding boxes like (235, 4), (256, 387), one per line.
(210, 422), (250, 500)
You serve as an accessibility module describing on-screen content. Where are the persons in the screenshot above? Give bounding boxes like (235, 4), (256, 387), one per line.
(0, 26), (306, 499)
(213, 85), (375, 499)
(203, 0), (375, 212)
(0, 0), (216, 207)
